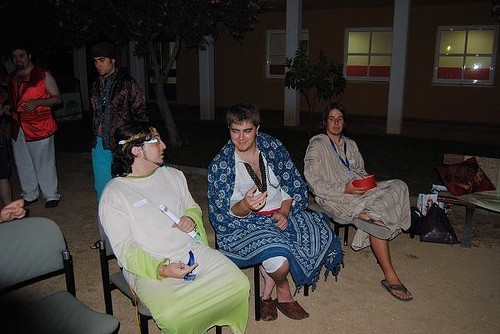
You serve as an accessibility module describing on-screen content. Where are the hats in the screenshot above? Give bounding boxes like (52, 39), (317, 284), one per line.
(92, 42), (114, 57)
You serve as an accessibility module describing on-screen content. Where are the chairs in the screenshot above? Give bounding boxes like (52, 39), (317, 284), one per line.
(0, 216), (120, 334)
(90, 215), (222, 334)
(301, 170), (357, 246)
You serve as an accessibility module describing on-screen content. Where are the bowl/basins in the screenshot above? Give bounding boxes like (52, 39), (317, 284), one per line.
(353, 175), (375, 191)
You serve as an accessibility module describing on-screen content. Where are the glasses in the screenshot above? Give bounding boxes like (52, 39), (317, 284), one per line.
(143, 138), (160, 144)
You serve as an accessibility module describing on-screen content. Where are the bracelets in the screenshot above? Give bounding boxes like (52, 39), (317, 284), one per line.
(162, 264), (167, 278)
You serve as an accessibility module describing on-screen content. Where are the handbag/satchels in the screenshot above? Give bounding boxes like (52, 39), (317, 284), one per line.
(401, 184), (459, 244)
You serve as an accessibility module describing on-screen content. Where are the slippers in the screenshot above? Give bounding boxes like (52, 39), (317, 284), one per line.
(353, 217), (391, 239)
(381, 279), (413, 301)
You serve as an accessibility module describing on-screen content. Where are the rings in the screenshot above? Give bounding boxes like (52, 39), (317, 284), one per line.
(259, 202), (261, 205)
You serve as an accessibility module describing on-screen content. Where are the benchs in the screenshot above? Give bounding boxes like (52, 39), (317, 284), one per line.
(437, 153), (500, 248)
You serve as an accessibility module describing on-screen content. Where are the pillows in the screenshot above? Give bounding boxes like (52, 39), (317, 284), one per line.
(434, 157), (496, 196)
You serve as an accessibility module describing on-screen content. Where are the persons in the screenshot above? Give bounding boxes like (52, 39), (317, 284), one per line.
(206, 102), (344, 321)
(0, 199), (26, 222)
(99, 116), (250, 334)
(0, 43), (61, 207)
(304, 103), (413, 301)
(90, 46), (145, 250)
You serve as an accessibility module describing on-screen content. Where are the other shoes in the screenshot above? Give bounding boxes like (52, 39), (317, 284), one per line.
(260, 296), (277, 321)
(24, 198), (38, 207)
(90, 238), (101, 250)
(45, 197), (61, 208)
(274, 298), (309, 320)
